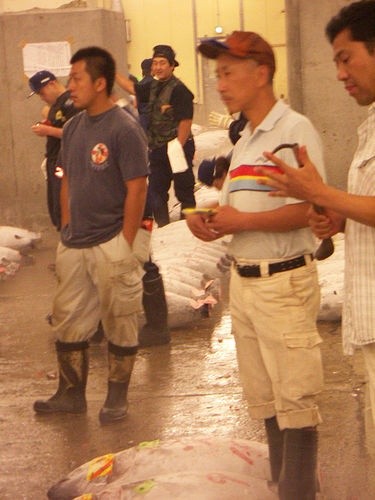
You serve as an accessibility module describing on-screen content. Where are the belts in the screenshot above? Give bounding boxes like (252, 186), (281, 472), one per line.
(235, 254), (313, 277)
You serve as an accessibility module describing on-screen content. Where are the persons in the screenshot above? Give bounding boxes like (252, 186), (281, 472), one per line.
(114, 44), (196, 227)
(185, 31), (327, 500)
(253, 0), (375, 460)
(32, 46), (150, 419)
(28, 71), (81, 230)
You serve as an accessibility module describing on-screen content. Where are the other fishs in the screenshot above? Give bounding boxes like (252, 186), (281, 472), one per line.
(48, 435), (320, 500)
(144, 122), (232, 326)
(1, 225), (42, 281)
(71, 469), (284, 500)
(315, 231), (350, 321)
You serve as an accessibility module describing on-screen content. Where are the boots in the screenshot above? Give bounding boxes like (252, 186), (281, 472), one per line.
(264, 415), (284, 482)
(99, 342), (140, 420)
(34, 340), (91, 413)
(278, 425), (318, 500)
(140, 274), (171, 346)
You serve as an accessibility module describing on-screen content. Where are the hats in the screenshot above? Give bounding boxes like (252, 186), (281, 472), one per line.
(154, 45), (179, 67)
(198, 157), (216, 186)
(198, 30), (274, 71)
(26, 70), (55, 98)
